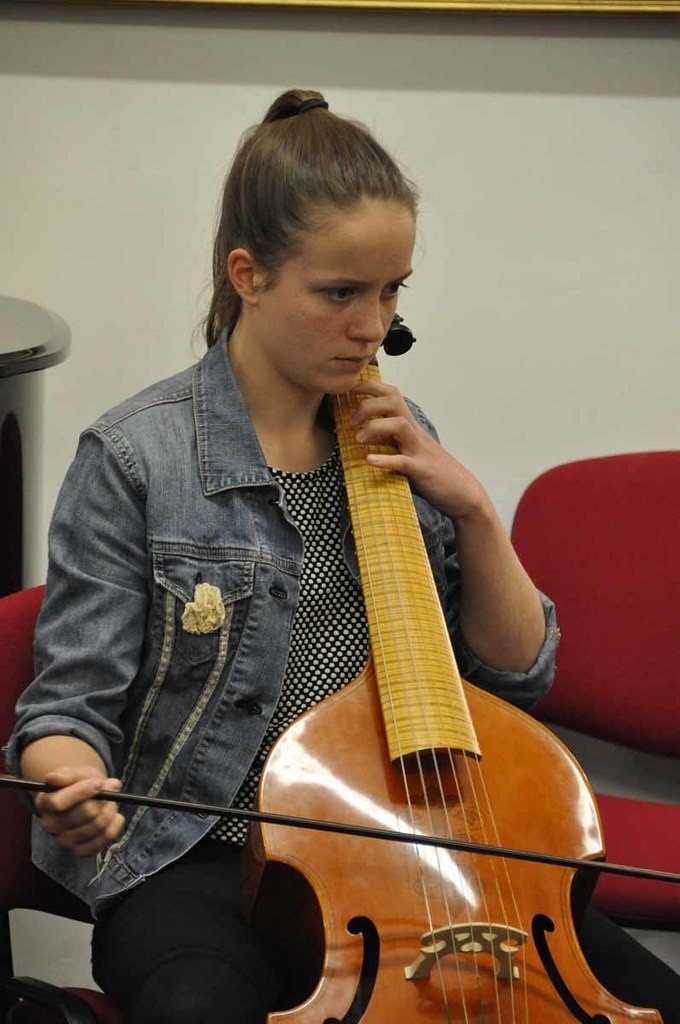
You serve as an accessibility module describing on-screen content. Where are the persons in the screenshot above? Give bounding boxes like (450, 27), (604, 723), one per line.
(4, 89), (679, 1024)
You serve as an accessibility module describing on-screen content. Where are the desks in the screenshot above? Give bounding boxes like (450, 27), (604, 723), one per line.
(0, 296), (70, 597)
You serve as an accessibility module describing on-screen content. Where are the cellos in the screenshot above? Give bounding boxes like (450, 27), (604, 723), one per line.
(253, 309), (666, 1022)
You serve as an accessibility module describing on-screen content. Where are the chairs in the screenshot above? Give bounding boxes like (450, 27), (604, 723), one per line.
(510, 450), (680, 930)
(0, 584), (98, 1024)
